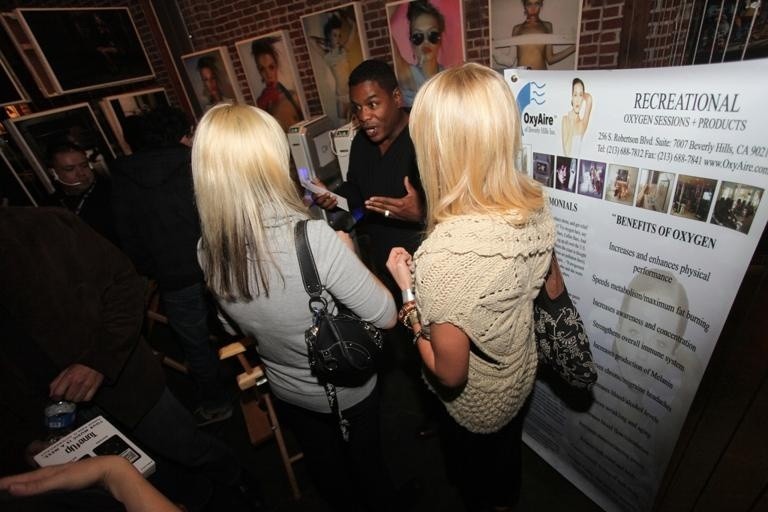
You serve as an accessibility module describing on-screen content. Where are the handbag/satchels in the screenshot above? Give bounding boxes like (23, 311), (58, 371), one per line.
(535, 254), (597, 388)
(306, 306), (383, 387)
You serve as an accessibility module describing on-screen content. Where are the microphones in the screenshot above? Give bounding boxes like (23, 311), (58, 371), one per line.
(50, 169), (81, 186)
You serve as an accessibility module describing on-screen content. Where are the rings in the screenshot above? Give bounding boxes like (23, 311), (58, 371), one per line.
(383, 208), (389, 218)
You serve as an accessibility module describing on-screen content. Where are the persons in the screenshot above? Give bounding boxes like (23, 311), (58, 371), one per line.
(383, 59), (559, 511)
(193, 54), (233, 109)
(508, 0), (576, 70)
(189, 102), (427, 512)
(248, 37), (302, 133)
(557, 157), (570, 189)
(308, 12), (358, 119)
(560, 78), (593, 156)
(0, 102), (242, 512)
(387, 0), (449, 103)
(560, 268), (692, 511)
(311, 56), (442, 439)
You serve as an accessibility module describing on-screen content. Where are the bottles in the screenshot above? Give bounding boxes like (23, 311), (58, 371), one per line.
(40, 383), (76, 453)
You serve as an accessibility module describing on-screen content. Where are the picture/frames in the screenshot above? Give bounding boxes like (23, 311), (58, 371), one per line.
(0, 7), (174, 207)
(384, 0), (467, 108)
(488, 0), (584, 76)
(179, 44), (246, 118)
(234, 28), (309, 133)
(298, 1), (370, 129)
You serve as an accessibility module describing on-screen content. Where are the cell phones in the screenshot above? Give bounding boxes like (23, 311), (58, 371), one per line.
(342, 205), (364, 232)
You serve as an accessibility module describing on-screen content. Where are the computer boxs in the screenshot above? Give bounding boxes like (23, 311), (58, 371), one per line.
(328, 119), (363, 182)
(287, 115), (338, 200)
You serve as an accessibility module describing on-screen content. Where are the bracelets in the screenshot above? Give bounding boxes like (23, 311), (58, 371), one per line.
(397, 289), (423, 345)
